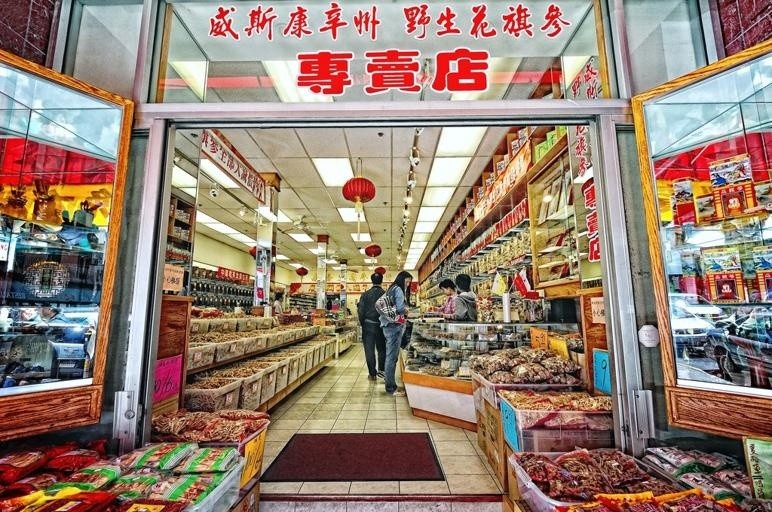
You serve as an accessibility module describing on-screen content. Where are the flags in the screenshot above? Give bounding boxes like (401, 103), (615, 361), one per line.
(491, 273), (505, 297)
(513, 270), (532, 297)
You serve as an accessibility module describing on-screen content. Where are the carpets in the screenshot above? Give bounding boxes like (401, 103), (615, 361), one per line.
(259, 432), (447, 482)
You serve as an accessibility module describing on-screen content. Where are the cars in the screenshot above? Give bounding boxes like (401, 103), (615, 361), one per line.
(668, 293), (772, 378)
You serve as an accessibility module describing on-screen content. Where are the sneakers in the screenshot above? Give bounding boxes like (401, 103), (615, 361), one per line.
(368, 370), (385, 379)
(386, 386), (405, 397)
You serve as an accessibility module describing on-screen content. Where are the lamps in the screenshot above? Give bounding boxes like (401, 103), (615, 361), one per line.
(397, 127), (423, 270)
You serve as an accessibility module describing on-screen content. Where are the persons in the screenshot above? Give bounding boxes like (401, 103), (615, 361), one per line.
(272, 292), (285, 314)
(326, 298), (332, 310)
(438, 273), (478, 322)
(427, 277), (457, 320)
(357, 271), (386, 381)
(332, 300), (339, 311)
(375, 270), (413, 397)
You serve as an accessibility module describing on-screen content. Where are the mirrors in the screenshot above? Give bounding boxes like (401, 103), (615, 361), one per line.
(630, 38), (772, 443)
(1, 47), (136, 446)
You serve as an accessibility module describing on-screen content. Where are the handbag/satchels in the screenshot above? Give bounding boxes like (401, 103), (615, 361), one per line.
(374, 285), (405, 324)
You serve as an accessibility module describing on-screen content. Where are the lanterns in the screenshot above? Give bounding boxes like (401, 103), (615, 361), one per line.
(374, 267), (386, 276)
(248, 245), (257, 260)
(296, 266), (308, 277)
(364, 243), (382, 258)
(342, 175), (376, 204)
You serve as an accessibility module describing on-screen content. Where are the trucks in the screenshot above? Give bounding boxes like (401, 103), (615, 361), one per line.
(47, 323), (90, 378)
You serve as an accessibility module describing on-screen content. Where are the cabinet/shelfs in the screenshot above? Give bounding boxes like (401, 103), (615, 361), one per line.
(161, 193), (196, 294)
(401, 125), (607, 433)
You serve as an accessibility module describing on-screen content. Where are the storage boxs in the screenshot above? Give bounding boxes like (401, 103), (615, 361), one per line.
(0, 408), (270, 512)
(181, 317), (355, 414)
(470, 326), (772, 512)
